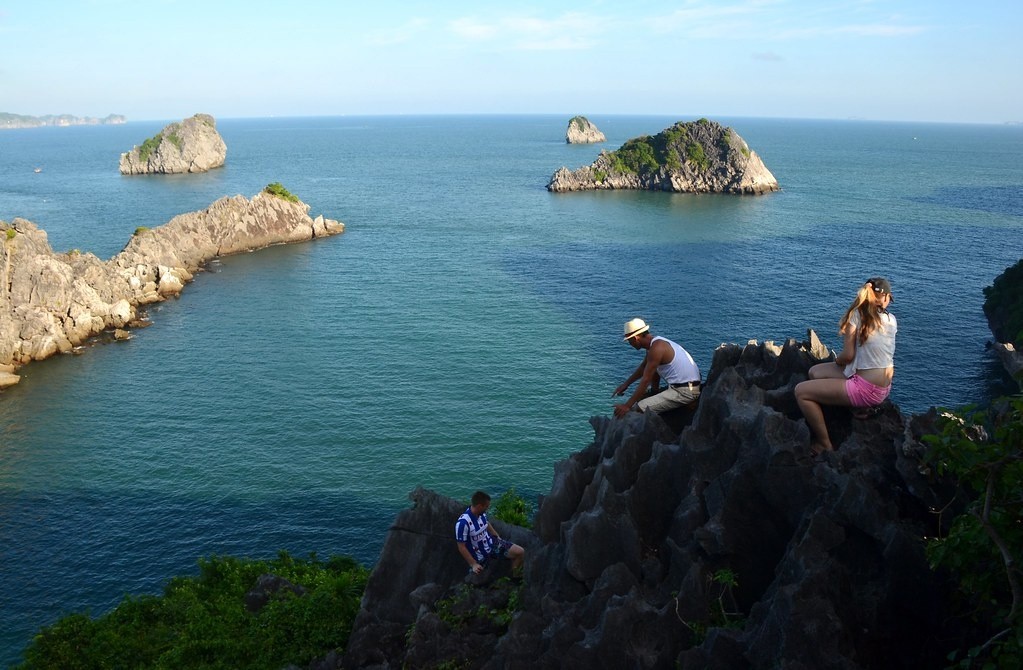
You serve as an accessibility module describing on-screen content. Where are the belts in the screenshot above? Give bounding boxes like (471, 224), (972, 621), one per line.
(671, 381), (700, 388)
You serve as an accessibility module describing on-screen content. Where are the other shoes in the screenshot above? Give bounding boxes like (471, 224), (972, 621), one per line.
(807, 448), (819, 457)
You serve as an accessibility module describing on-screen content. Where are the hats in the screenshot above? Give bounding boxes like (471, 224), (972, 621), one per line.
(867, 278), (894, 303)
(622, 318), (649, 341)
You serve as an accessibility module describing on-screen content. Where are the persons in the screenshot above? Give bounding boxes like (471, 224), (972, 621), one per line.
(794, 277), (897, 457)
(455, 491), (525, 584)
(609, 318), (701, 420)
